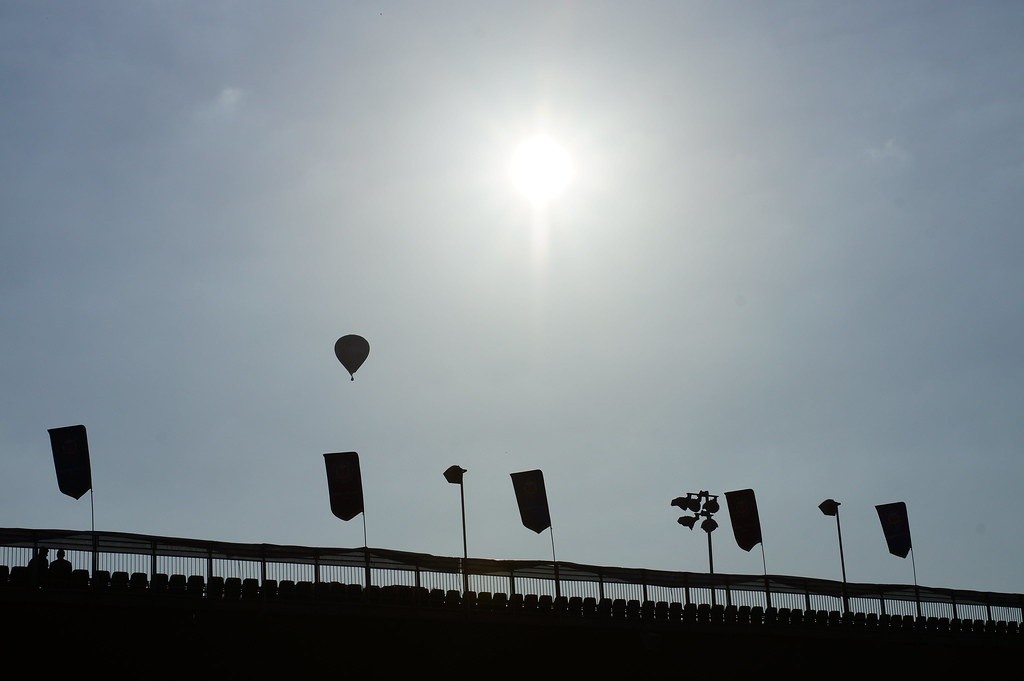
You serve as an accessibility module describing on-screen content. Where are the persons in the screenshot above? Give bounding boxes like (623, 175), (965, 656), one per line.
(50, 550), (72, 573)
(28, 547), (49, 573)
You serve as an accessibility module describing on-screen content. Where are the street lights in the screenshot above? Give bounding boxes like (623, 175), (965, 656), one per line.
(818, 499), (847, 585)
(443, 466), (469, 559)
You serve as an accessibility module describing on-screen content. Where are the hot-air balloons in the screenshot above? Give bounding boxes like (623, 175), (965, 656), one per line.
(333, 334), (371, 383)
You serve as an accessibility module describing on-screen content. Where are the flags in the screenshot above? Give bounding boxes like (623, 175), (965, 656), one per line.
(725, 489), (761, 551)
(875, 502), (912, 558)
(511, 469), (551, 533)
(323, 452), (364, 521)
(49, 424), (91, 500)
